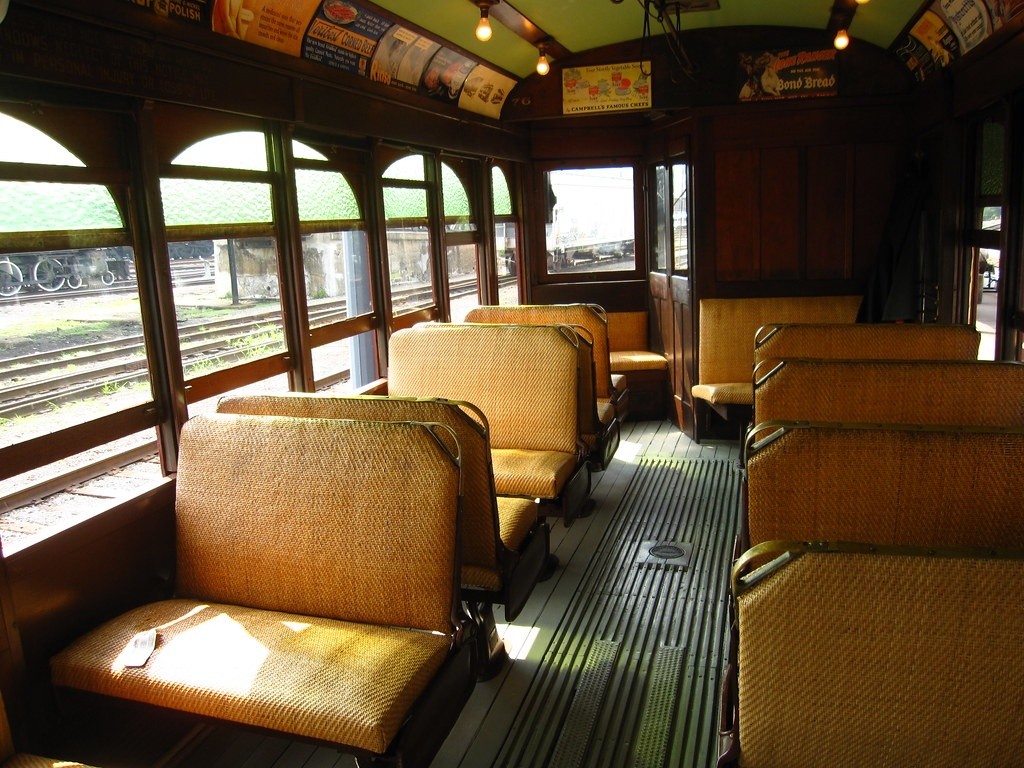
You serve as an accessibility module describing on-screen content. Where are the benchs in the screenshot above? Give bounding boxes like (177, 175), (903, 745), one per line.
(716, 540), (1024, 768)
(216, 392), (544, 592)
(740, 419), (1024, 547)
(753, 357), (1024, 427)
(754, 323), (981, 360)
(465, 303), (617, 405)
(597, 311), (667, 372)
(387, 324), (581, 497)
(412, 320), (607, 449)
(50, 414), (465, 756)
(691, 296), (863, 444)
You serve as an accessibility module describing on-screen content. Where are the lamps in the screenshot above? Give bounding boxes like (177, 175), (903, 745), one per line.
(469, 0), (503, 43)
(830, 14), (852, 50)
(532, 36), (555, 75)
